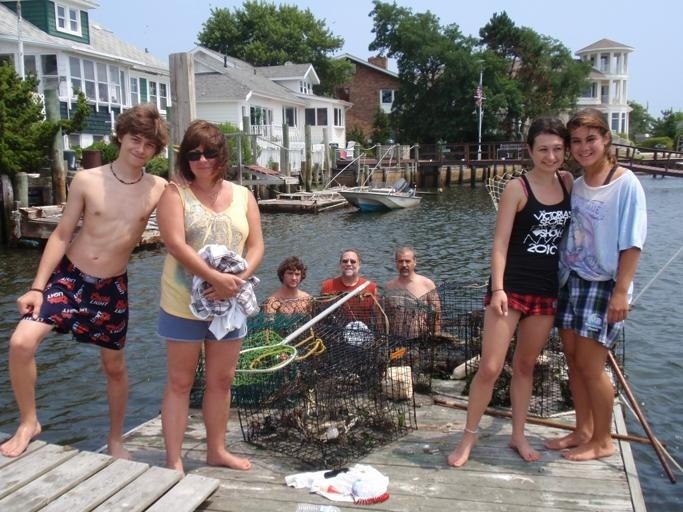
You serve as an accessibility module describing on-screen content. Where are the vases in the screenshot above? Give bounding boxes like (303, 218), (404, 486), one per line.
(81, 150), (102, 169)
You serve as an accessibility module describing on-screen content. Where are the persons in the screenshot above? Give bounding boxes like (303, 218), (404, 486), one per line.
(447, 118), (574, 467)
(155, 121), (263, 475)
(318, 249), (384, 334)
(265, 256), (311, 313)
(542, 108), (647, 461)
(0, 101), (171, 460)
(382, 246), (445, 344)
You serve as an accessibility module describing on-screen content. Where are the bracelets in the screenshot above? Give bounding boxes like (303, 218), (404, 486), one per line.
(26, 288), (43, 294)
(489, 288), (504, 294)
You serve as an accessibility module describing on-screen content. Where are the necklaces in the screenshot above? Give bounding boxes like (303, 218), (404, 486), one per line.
(109, 161), (145, 185)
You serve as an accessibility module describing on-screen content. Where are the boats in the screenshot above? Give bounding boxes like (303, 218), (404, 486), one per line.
(338, 143), (424, 213)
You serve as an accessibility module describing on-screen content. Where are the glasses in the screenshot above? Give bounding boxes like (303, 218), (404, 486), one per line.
(342, 258), (358, 265)
(182, 148), (221, 162)
